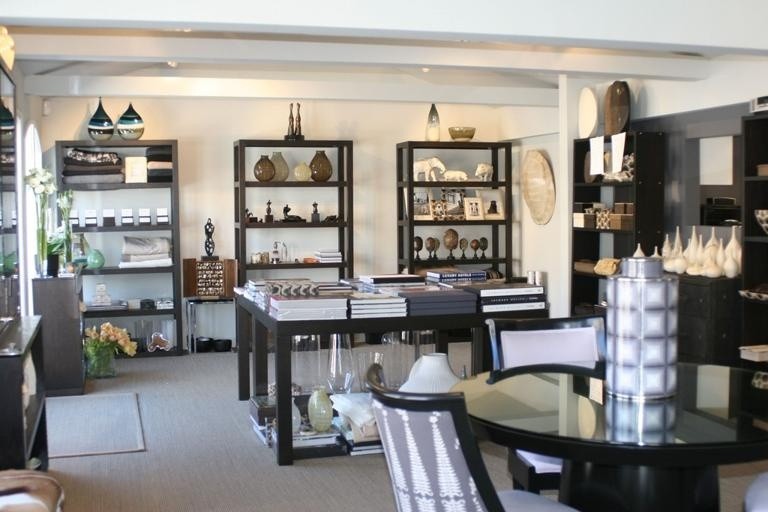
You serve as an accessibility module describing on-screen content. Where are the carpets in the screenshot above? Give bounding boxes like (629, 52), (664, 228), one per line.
(45, 392), (147, 458)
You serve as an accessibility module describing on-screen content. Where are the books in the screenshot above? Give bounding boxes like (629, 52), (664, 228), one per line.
(234, 267), (544, 322)
(271, 417), (384, 456)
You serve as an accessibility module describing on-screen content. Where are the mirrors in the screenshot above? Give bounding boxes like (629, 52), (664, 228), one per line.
(0, 60), (20, 338)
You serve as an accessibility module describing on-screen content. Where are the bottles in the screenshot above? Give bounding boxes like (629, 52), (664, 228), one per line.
(116, 103), (145, 140)
(251, 149), (334, 183)
(281, 240), (287, 262)
(271, 242), (280, 264)
(88, 96), (114, 141)
(633, 223), (742, 280)
(86, 248), (105, 269)
(289, 367), (468, 431)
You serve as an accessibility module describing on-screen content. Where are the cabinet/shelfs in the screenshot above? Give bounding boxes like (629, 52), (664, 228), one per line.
(233, 138), (353, 352)
(0, 313), (49, 472)
(397, 141), (511, 281)
(55, 138), (183, 358)
(0, 117), (18, 315)
(739, 113), (768, 430)
(570, 132), (663, 319)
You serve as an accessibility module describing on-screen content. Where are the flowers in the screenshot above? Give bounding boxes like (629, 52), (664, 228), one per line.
(56, 189), (74, 260)
(24, 168), (57, 261)
(83, 323), (138, 368)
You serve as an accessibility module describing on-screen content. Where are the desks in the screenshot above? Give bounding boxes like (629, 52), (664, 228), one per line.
(186, 300), (234, 354)
(450, 361), (768, 512)
(237, 295), (550, 467)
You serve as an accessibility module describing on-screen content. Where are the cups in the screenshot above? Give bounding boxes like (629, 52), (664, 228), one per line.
(250, 252), (270, 264)
(325, 332), (356, 396)
(133, 319), (177, 354)
(303, 258), (315, 263)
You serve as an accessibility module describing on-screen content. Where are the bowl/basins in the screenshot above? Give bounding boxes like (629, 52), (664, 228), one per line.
(753, 209), (768, 233)
(447, 126), (477, 141)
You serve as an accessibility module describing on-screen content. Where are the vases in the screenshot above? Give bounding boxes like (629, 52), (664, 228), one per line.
(33, 194), (49, 278)
(60, 210), (72, 264)
(87, 355), (118, 378)
(87, 249), (104, 268)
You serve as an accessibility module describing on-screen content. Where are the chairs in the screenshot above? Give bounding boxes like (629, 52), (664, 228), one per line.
(365, 363), (580, 512)
(485, 315), (606, 495)
